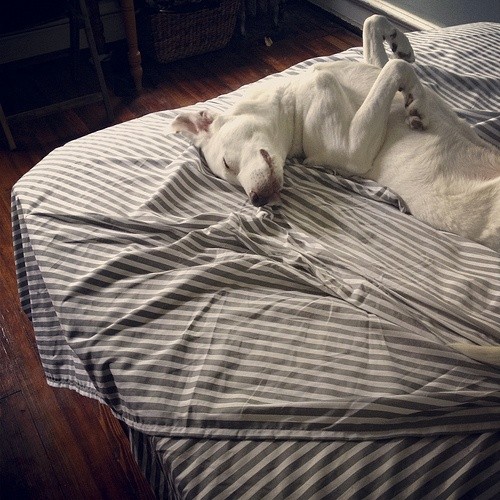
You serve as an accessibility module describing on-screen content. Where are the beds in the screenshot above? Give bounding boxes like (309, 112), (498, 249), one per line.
(7, 17), (500, 500)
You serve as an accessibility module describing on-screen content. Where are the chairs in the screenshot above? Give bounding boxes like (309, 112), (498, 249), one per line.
(0, 0), (114, 151)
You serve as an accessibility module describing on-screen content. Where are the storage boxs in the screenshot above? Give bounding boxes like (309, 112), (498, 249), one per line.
(146, 0), (241, 64)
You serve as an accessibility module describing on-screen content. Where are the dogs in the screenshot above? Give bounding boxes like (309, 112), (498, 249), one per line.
(172, 14), (500, 254)
